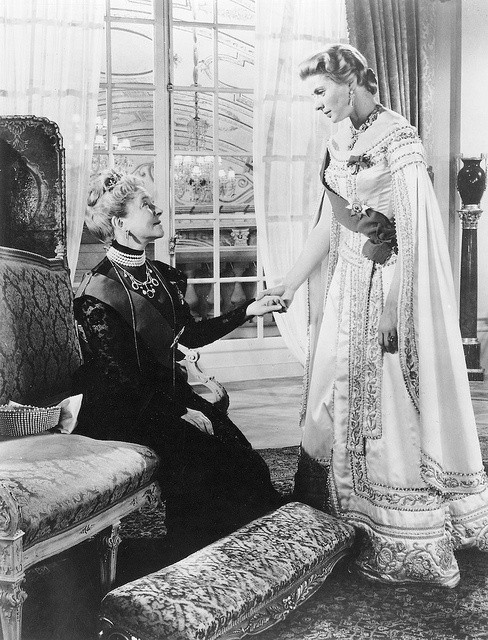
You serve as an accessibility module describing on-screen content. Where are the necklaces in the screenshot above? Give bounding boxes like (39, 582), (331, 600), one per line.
(104, 248), (162, 300)
(346, 102), (387, 151)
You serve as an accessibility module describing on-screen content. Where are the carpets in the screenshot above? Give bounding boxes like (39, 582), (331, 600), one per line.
(20, 424), (488, 640)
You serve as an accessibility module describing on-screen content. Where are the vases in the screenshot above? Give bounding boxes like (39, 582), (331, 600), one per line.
(456, 154), (488, 210)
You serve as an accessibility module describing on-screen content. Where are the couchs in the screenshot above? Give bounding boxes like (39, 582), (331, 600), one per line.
(0, 242), (230, 640)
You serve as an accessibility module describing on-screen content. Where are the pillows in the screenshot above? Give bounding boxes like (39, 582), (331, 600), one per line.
(101, 502), (355, 640)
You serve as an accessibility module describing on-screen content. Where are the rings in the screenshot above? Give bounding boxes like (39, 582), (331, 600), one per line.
(387, 336), (395, 344)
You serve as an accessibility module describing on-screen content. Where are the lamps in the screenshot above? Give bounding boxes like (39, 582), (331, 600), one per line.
(173, 16), (237, 203)
(93, 116), (131, 174)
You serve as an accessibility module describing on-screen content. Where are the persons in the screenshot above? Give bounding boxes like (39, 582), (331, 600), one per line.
(72, 168), (286, 535)
(252, 43), (488, 587)
